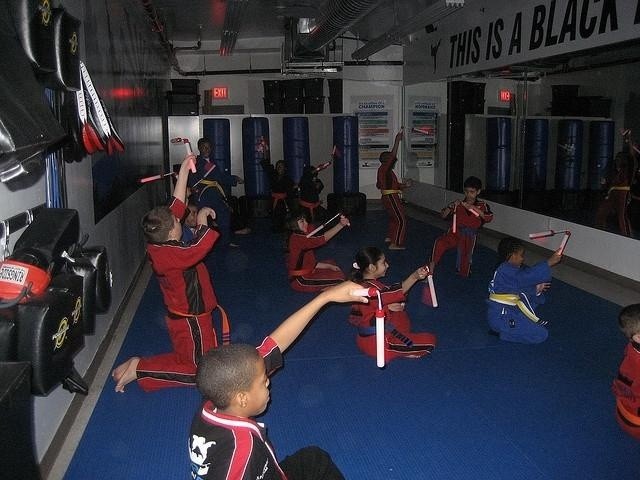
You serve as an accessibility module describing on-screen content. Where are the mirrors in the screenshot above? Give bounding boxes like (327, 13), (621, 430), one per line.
(445, 37), (640, 240)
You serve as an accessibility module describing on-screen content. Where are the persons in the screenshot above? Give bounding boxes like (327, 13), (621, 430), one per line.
(348, 246), (436, 364)
(282, 209), (349, 293)
(431, 176), (493, 280)
(376, 133), (411, 250)
(613, 303), (640, 443)
(111, 153), (230, 393)
(187, 280), (368, 480)
(485, 236), (562, 344)
(594, 128), (638, 237)
(179, 137), (331, 251)
